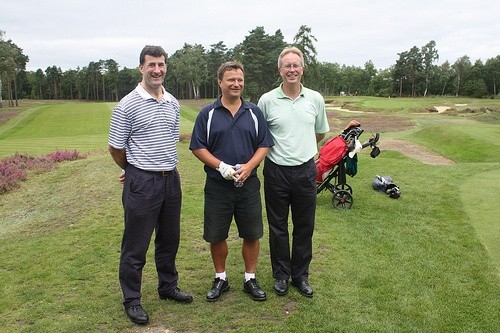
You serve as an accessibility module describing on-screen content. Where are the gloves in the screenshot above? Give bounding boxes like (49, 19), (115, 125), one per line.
(215, 161), (240, 182)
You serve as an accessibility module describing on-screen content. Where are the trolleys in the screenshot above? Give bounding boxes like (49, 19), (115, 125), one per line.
(314, 120), (381, 211)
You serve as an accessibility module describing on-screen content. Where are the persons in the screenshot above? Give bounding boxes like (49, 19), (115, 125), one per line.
(108, 46), (193, 324)
(258, 47), (329, 297)
(189, 61), (275, 301)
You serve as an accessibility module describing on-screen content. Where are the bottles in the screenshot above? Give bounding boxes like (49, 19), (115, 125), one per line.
(234, 164), (243, 187)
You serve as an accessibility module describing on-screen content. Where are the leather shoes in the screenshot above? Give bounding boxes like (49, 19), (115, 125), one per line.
(243, 278), (266, 300)
(159, 287), (193, 303)
(205, 276), (230, 301)
(291, 280), (313, 297)
(274, 280), (288, 296)
(123, 304), (149, 323)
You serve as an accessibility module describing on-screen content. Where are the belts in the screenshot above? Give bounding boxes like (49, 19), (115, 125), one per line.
(140, 169), (176, 177)
